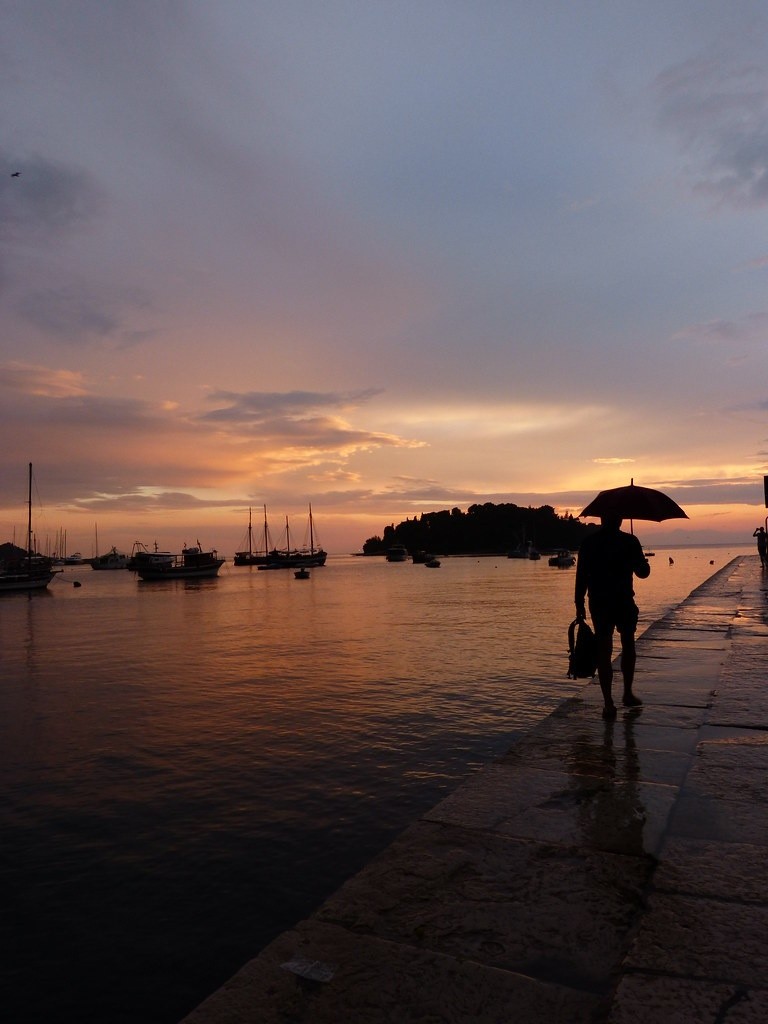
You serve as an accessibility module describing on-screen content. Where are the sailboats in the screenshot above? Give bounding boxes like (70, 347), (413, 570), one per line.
(90, 523), (131, 570)
(262, 503), (327, 568)
(0, 462), (63, 591)
(385, 523), (408, 562)
(52, 526), (64, 566)
(233, 504), (272, 566)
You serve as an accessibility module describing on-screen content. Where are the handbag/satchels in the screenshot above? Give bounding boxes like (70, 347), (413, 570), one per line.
(567, 617), (601, 681)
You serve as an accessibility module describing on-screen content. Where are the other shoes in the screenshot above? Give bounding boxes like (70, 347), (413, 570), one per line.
(622, 697), (644, 707)
(601, 707), (618, 721)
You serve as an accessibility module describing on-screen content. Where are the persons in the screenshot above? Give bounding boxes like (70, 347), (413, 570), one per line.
(574, 516), (650, 721)
(753, 527), (768, 567)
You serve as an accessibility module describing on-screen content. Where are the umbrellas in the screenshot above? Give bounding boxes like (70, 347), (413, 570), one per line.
(577, 479), (690, 535)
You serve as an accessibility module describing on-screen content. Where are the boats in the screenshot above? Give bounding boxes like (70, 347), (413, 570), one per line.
(528, 551), (541, 560)
(548, 548), (576, 566)
(126, 540), (172, 571)
(66, 555), (82, 565)
(134, 540), (225, 581)
(645, 553), (655, 556)
(413, 551), (435, 564)
(294, 569), (310, 578)
(425, 559), (440, 568)
